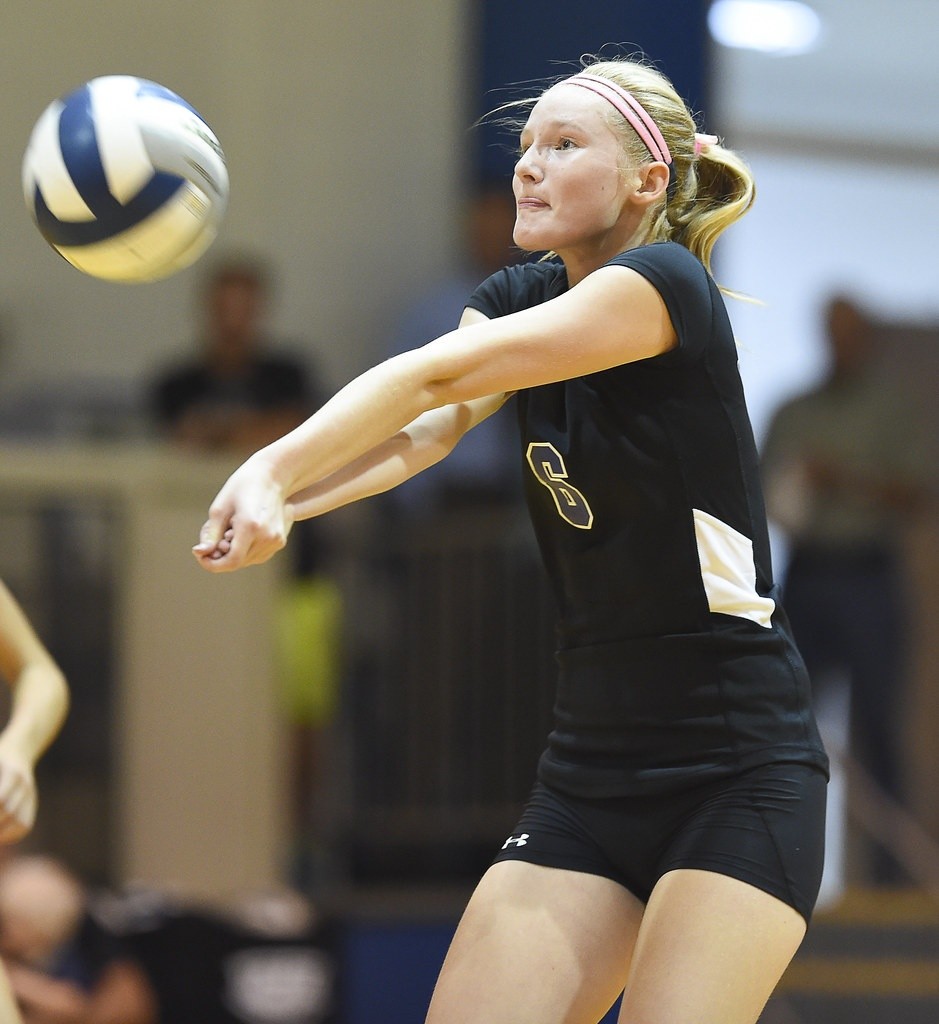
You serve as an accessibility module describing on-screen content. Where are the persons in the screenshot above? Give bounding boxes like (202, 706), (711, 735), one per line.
(192, 60), (832, 1023)
(0, 556), (163, 1024)
(756, 318), (937, 893)
(143, 244), (342, 900)
(355, 74), (637, 1023)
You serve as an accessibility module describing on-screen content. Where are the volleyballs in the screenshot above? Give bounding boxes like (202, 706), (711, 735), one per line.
(19, 73), (232, 285)
